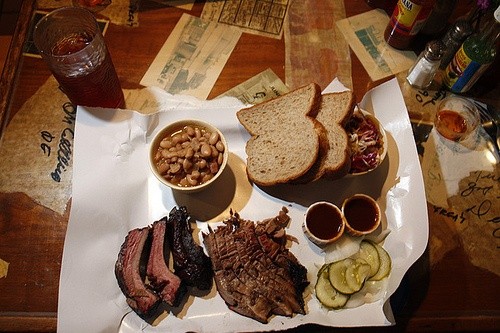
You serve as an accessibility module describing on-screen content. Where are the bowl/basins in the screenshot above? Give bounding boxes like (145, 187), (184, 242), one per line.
(147, 118), (228, 193)
(341, 108), (387, 178)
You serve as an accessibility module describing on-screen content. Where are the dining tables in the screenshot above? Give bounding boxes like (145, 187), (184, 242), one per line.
(0, 0), (500, 333)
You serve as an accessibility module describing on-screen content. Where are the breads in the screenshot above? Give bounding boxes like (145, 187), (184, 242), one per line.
(292, 91), (357, 185)
(236, 83), (329, 186)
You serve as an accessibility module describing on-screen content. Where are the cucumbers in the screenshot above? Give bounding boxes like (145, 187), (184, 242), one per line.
(315, 239), (392, 308)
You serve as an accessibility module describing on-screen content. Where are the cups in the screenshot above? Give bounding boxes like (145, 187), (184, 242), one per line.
(434, 96), (481, 139)
(33, 7), (126, 116)
(302, 201), (345, 247)
(338, 194), (381, 237)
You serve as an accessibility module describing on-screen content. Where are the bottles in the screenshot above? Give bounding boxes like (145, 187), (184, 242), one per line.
(405, 39), (447, 90)
(441, 4), (500, 95)
(383, 0), (439, 50)
(439, 0), (492, 70)
